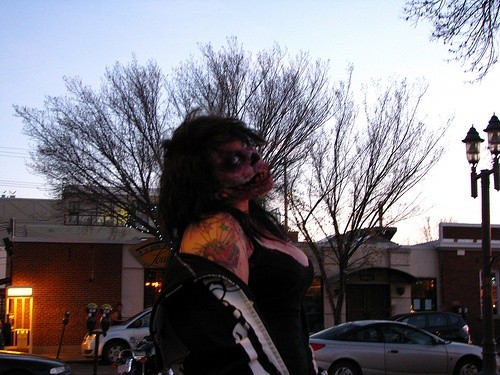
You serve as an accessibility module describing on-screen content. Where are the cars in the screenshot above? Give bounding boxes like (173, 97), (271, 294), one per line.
(367, 310), (472, 345)
(310, 319), (483, 375)
(0, 353), (71, 375)
(81, 305), (155, 366)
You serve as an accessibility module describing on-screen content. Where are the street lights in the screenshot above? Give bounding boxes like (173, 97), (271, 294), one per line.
(461, 111), (500, 375)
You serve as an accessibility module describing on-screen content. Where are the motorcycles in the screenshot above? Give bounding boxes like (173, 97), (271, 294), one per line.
(113, 333), (168, 375)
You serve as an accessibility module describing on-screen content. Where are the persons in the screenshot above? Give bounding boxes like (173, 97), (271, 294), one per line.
(148, 108), (317, 375)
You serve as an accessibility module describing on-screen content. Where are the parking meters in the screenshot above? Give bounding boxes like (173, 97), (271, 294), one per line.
(86, 304), (112, 375)
